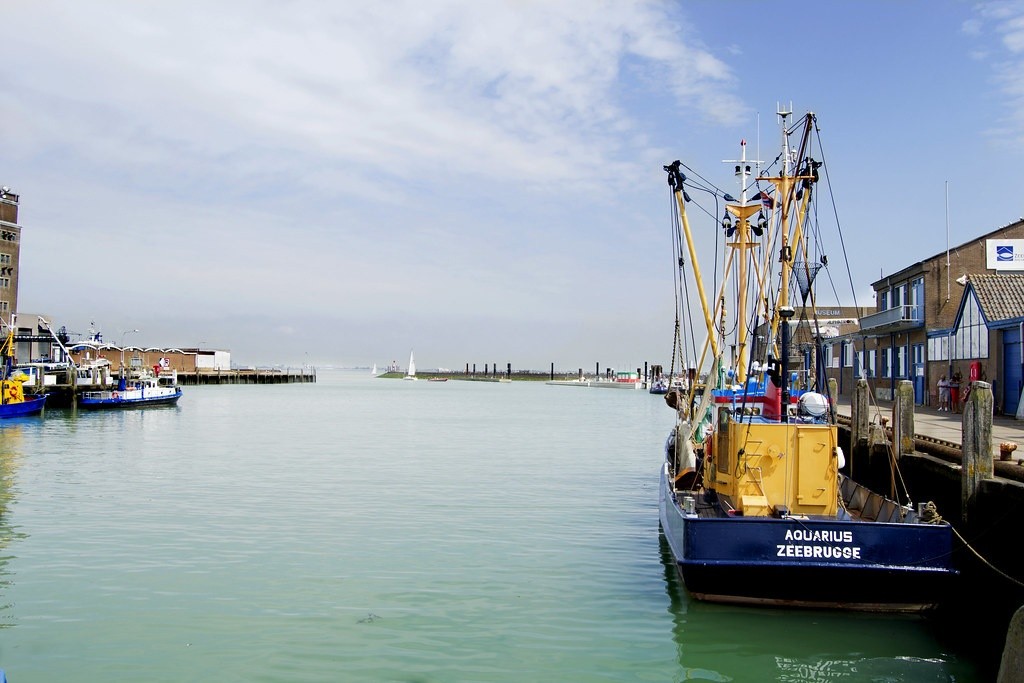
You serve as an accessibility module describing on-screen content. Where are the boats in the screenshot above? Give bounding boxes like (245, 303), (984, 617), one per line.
(649, 100), (961, 614)
(0, 314), (182, 419)
(430, 377), (448, 382)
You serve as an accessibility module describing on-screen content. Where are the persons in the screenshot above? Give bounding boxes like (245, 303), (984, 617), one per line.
(950, 372), (967, 414)
(937, 374), (950, 412)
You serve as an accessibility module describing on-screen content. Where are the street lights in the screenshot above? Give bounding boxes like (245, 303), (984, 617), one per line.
(196, 342), (206, 367)
(123, 330), (139, 362)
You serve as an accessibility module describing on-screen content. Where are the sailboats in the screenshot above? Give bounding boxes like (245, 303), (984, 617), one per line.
(403, 351), (417, 380)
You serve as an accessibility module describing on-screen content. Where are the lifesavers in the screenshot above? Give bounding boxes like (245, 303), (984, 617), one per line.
(112, 392), (118, 399)
(10, 387), (18, 397)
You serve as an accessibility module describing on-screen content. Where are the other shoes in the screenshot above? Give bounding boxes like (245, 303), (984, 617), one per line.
(945, 408), (947, 411)
(937, 408), (944, 412)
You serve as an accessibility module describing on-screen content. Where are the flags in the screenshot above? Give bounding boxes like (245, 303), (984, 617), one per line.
(760, 190), (781, 209)
(89, 332), (102, 342)
